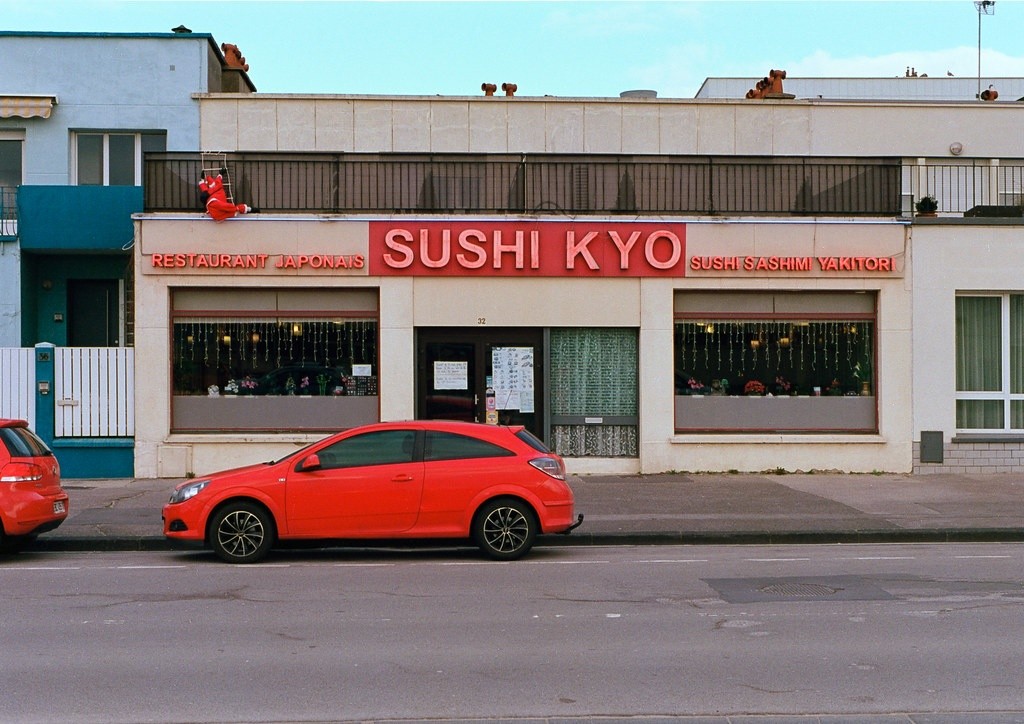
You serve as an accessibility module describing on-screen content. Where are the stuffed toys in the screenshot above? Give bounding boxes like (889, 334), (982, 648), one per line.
(198, 168), (261, 222)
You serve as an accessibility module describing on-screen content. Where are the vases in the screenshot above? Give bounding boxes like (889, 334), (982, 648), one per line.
(748, 392), (761, 396)
(775, 384), (785, 394)
(828, 393), (833, 396)
(304, 387), (308, 395)
(319, 383), (325, 395)
(690, 389), (703, 395)
(861, 381), (871, 396)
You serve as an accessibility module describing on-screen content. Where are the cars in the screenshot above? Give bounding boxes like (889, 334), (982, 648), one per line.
(424, 394), (480, 422)
(0, 416), (69, 562)
(161, 419), (584, 564)
(226, 364), (348, 396)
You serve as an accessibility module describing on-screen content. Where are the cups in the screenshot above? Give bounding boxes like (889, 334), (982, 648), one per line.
(814, 386), (820, 397)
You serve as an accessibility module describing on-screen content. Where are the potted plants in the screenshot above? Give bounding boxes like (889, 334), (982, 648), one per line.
(915, 197), (937, 217)
(285, 377), (296, 395)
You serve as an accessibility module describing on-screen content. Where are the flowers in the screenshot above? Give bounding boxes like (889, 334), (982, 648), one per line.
(300, 377), (309, 388)
(239, 375), (258, 395)
(825, 378), (841, 396)
(315, 370), (332, 395)
(775, 375), (790, 390)
(853, 362), (867, 383)
(224, 376), (238, 394)
(744, 380), (765, 393)
(688, 376), (704, 389)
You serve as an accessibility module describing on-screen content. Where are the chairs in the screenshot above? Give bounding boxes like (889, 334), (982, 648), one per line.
(401, 434), (412, 454)
